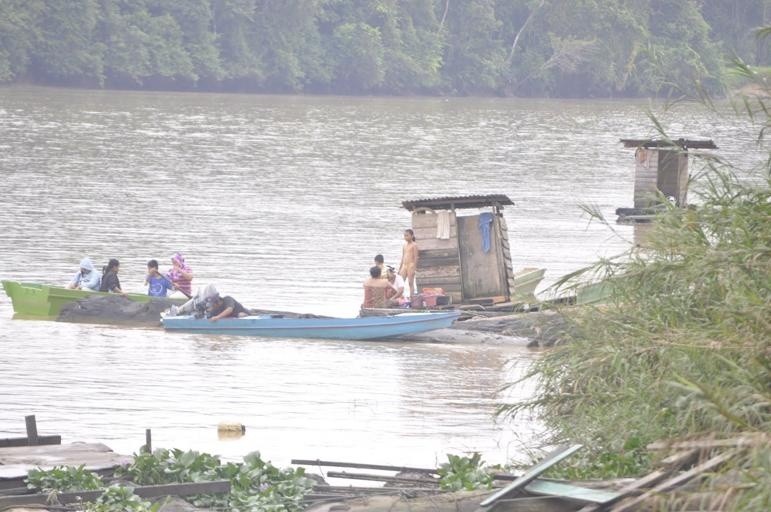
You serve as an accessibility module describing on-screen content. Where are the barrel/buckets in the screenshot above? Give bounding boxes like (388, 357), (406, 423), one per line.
(411, 294), (423, 308)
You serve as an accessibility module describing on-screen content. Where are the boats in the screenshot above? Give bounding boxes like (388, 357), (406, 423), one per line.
(512, 264), (547, 296)
(359, 194), (577, 318)
(162, 309), (461, 341)
(1, 279), (185, 326)
(616, 138), (718, 222)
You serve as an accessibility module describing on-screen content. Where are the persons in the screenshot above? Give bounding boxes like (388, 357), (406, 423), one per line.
(363, 266), (401, 308)
(65, 257), (102, 293)
(397, 228), (419, 302)
(202, 283), (251, 322)
(99, 258), (122, 294)
(166, 252), (193, 299)
(385, 266), (405, 306)
(143, 260), (179, 297)
(375, 254), (391, 280)
